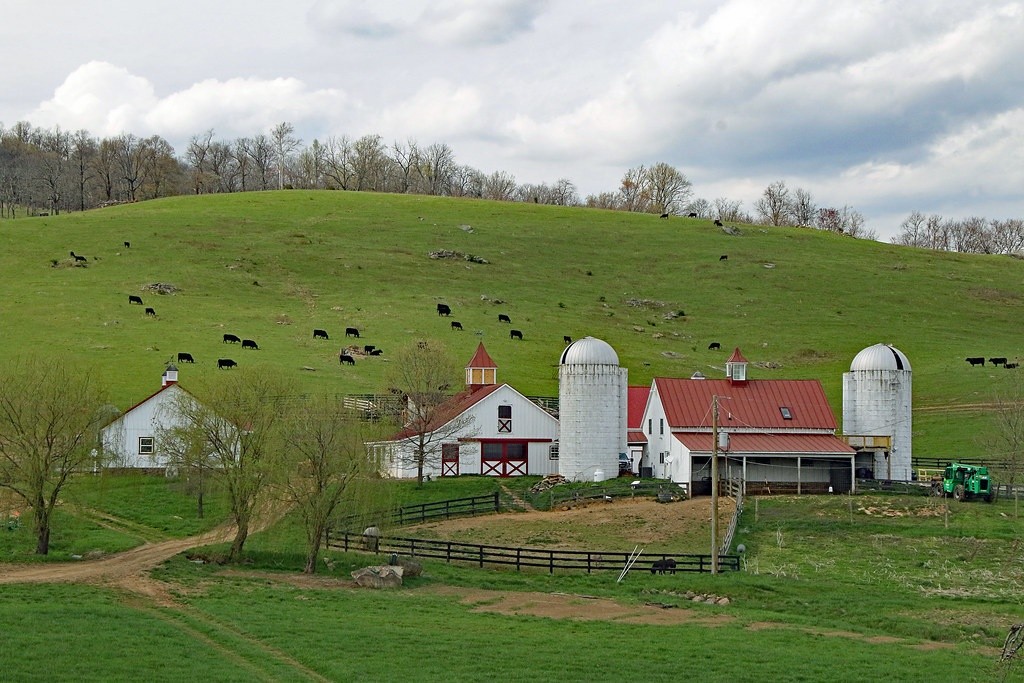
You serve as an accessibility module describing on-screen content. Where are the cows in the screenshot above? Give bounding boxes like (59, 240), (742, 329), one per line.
(719, 256), (727, 261)
(129, 296), (155, 315)
(965, 358), (1015, 369)
(346, 328), (360, 337)
(564, 336), (571, 344)
(223, 334), (258, 349)
(651, 559), (676, 575)
(340, 355), (355, 365)
(365, 346), (383, 355)
(40, 212), (48, 216)
(498, 314), (523, 340)
(124, 242), (129, 247)
(313, 330), (328, 339)
(178, 353), (194, 364)
(70, 251), (87, 262)
(708, 342), (720, 349)
(437, 304), (462, 331)
(660, 213), (723, 227)
(217, 359), (237, 370)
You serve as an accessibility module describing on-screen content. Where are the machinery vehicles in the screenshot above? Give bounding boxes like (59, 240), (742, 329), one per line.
(930, 461), (995, 506)
